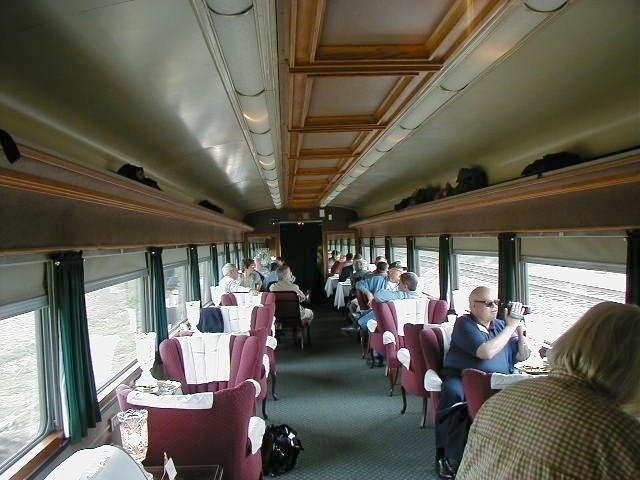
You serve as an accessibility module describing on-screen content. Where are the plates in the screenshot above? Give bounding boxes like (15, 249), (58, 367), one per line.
(519, 362), (551, 375)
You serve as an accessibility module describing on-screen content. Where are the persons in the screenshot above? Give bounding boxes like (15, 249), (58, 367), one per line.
(355, 260), (407, 338)
(240, 258), (263, 293)
(264, 263), (278, 289)
(221, 263), (259, 295)
(330, 249), (367, 282)
(375, 257), (387, 265)
(434, 287), (530, 464)
(375, 272), (418, 302)
(269, 266), (313, 339)
(456, 303), (638, 475)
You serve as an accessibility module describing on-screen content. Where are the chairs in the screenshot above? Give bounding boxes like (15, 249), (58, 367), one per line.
(270, 291), (311, 350)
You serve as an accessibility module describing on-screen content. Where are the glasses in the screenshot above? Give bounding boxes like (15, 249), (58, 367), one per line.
(473, 299), (500, 307)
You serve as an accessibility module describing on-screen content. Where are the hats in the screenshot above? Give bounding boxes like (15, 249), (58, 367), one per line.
(388, 261), (409, 270)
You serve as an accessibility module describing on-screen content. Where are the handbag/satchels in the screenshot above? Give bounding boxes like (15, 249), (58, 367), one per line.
(262, 423), (304, 474)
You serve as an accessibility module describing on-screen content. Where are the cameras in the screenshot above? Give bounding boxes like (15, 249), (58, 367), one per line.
(500, 301), (532, 314)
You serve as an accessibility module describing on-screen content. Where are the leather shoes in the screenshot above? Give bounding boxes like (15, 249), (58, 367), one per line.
(435, 456), (456, 480)
(341, 326), (359, 337)
(366, 358), (384, 368)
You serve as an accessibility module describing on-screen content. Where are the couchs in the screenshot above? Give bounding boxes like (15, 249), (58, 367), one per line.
(357, 289), (371, 359)
(382, 299), (447, 397)
(265, 340), (278, 400)
(420, 327), (457, 423)
(196, 305), (273, 337)
(160, 336), (261, 396)
(370, 299), (389, 368)
(178, 327), (268, 419)
(460, 367), (490, 421)
(116, 379), (262, 480)
(399, 323), (440, 428)
(221, 292), (275, 315)
